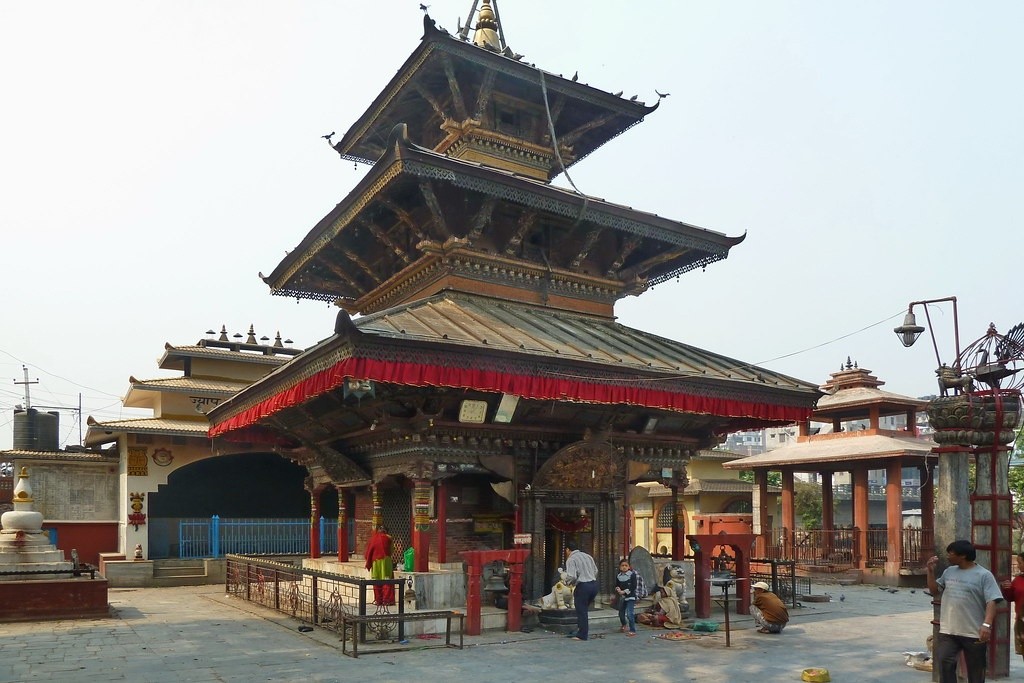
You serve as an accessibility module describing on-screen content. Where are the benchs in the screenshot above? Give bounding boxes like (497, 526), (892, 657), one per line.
(341, 611), (464, 658)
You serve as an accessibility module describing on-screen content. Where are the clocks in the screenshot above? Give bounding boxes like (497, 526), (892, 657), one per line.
(459, 400), (487, 424)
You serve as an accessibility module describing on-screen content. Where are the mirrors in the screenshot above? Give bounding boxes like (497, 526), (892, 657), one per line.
(492, 392), (522, 424)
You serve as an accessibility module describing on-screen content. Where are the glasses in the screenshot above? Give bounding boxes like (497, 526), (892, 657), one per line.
(754, 588), (762, 590)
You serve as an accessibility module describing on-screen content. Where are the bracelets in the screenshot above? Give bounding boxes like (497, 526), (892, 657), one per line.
(981, 622), (993, 629)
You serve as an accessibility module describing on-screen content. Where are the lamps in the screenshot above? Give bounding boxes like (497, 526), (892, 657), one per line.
(660, 465), (674, 479)
(895, 313), (926, 348)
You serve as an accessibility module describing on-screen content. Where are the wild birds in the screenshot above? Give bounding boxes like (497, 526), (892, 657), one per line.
(824, 586), (931, 603)
(419, 3), (671, 102)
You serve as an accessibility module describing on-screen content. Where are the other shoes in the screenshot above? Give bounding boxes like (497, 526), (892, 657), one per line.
(620, 625), (628, 632)
(572, 618), (582, 641)
(757, 627), (770, 633)
(626, 632), (637, 637)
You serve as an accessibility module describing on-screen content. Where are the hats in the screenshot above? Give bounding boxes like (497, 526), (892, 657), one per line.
(751, 582), (769, 591)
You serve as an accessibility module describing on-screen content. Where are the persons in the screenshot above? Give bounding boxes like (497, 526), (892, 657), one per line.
(614, 559), (637, 637)
(1001, 552), (1024, 661)
(749, 581), (790, 634)
(926, 539), (1004, 683)
(649, 586), (681, 627)
(365, 524), (396, 606)
(564, 543), (599, 640)
